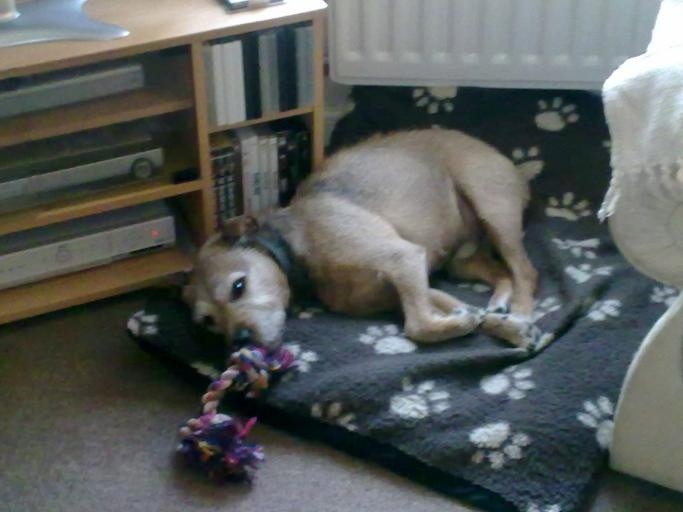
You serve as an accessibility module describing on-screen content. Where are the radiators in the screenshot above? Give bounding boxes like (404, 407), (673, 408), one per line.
(329, 1), (683, 90)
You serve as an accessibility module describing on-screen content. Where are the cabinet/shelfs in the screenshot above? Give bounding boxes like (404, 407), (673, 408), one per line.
(0, 0), (328, 326)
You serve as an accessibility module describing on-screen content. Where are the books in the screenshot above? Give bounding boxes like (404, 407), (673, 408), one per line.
(221, 0), (286, 15)
(203, 24), (314, 232)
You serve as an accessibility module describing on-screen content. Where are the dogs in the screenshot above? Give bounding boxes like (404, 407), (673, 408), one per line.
(181, 129), (544, 351)
